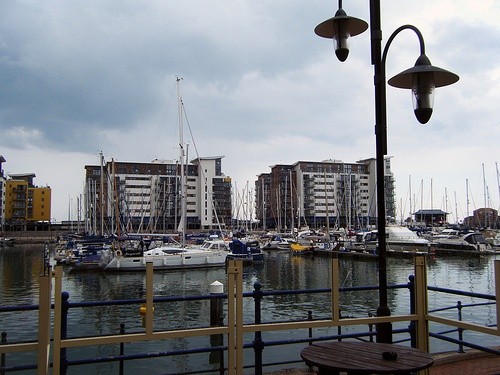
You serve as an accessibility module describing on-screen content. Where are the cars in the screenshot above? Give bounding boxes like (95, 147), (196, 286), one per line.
(481, 225), (500, 248)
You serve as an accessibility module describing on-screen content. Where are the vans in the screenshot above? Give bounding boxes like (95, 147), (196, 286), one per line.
(438, 229), (459, 238)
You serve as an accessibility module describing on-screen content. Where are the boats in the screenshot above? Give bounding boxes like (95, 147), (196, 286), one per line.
(50, 225), (430, 271)
(430, 231), (492, 253)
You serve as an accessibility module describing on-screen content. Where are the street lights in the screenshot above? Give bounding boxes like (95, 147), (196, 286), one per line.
(313, 0), (462, 340)
